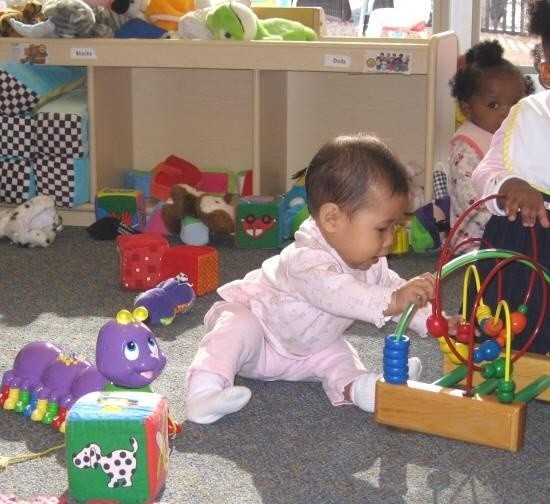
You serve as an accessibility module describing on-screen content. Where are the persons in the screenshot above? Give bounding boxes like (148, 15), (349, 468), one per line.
(450, 0), (550, 356)
(442, 38), (529, 256)
(182, 134), (481, 425)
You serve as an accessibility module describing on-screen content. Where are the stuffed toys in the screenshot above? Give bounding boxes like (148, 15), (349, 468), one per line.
(1, 194), (62, 249)
(0, 0), (318, 41)
(0, 62), (311, 504)
(0, 1), (318, 41)
(389, 159), (451, 257)
(163, 182), (235, 232)
